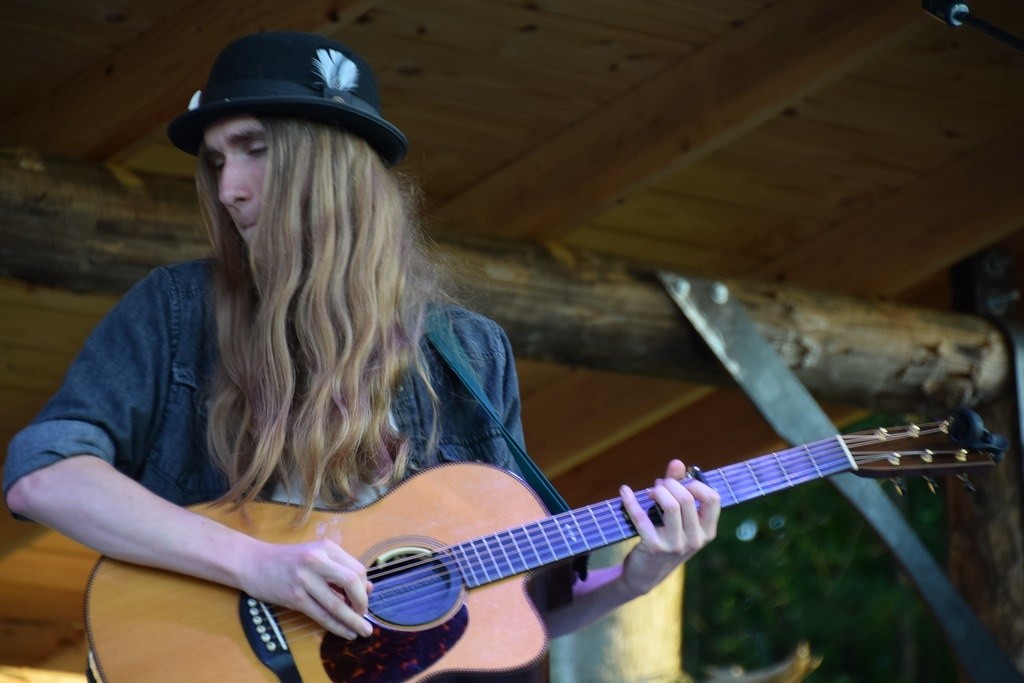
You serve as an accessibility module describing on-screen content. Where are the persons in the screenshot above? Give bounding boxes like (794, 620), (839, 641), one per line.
(1, 31), (721, 682)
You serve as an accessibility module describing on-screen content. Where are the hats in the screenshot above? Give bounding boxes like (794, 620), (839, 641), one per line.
(165, 30), (410, 170)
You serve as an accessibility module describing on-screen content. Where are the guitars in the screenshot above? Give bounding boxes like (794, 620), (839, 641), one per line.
(80, 410), (1009, 683)
